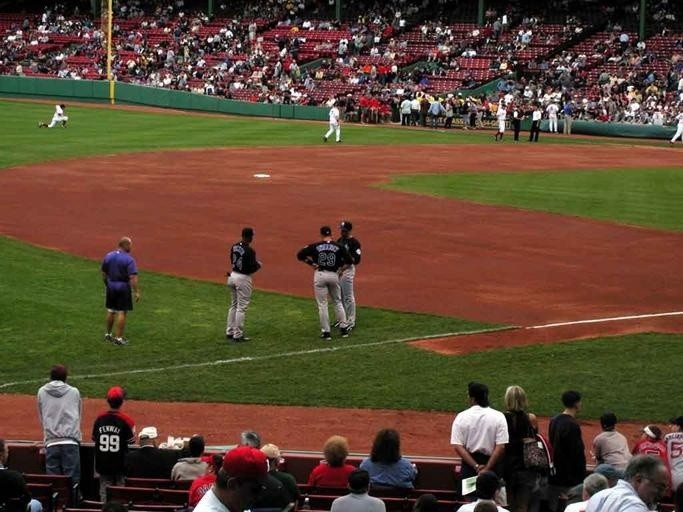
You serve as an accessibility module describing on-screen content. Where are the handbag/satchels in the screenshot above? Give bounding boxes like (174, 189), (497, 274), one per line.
(523, 437), (549, 470)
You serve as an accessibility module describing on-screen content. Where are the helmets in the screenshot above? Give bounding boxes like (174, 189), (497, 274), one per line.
(338, 219), (352, 230)
(320, 226), (332, 236)
(241, 227), (254, 237)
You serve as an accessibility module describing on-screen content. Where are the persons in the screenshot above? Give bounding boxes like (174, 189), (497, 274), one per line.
(36, 364), (85, 499)
(92, 384), (136, 503)
(449, 379), (683, 512)
(296, 224), (350, 341)
(0, 0), (682, 145)
(99, 235), (141, 346)
(169, 425), (418, 511)
(0, 438), (43, 511)
(224, 225), (264, 341)
(332, 220), (362, 330)
(138, 426), (161, 449)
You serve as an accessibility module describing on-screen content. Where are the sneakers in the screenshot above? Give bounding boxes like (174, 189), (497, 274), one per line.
(104, 333), (130, 345)
(226, 334), (251, 342)
(320, 320), (353, 341)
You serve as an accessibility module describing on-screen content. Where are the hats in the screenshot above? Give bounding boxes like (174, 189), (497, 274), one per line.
(200, 454), (222, 467)
(50, 364), (67, 382)
(138, 426), (158, 440)
(467, 382), (489, 408)
(107, 386), (128, 403)
(668, 416), (683, 425)
(223, 446), (284, 489)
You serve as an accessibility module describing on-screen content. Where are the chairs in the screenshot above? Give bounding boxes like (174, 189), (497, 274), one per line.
(4, 439), (674, 512)
(0, 18), (683, 105)
